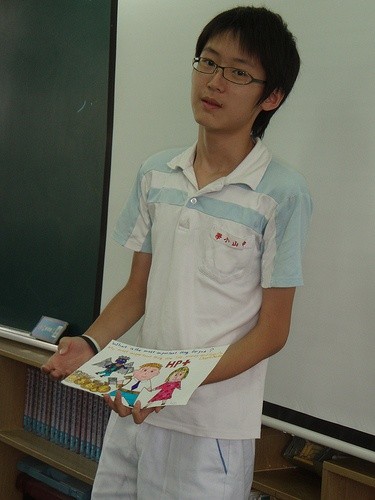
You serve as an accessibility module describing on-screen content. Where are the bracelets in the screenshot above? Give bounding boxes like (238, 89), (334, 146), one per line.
(80, 333), (102, 354)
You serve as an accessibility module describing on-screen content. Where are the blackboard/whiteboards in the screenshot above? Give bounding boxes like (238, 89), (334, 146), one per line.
(0, 0), (119, 355)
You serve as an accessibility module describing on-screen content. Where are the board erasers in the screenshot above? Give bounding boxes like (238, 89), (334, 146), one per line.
(31, 315), (69, 344)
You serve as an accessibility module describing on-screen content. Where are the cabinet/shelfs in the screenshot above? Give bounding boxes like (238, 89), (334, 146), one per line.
(0, 338), (375, 500)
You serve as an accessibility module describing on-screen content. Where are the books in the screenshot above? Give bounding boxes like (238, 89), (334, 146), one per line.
(18, 362), (117, 500)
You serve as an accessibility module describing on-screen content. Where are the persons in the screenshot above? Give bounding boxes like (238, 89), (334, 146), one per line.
(44, 5), (314, 500)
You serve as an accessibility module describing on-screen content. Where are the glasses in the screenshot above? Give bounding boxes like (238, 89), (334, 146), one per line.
(192, 56), (265, 85)
